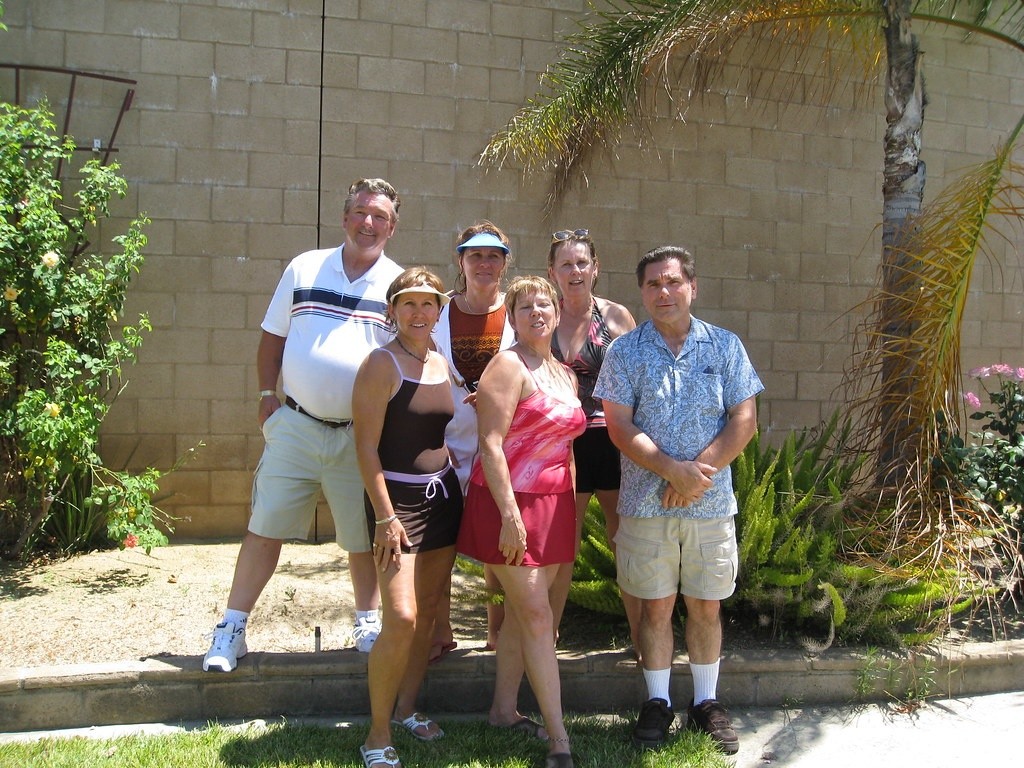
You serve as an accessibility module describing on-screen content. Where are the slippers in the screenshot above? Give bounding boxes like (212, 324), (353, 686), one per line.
(428, 640), (458, 663)
(361, 745), (402, 768)
(391, 712), (445, 741)
(544, 748), (574, 768)
(488, 715), (549, 742)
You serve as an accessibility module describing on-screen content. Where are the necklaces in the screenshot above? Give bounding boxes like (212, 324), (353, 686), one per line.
(389, 331), (445, 374)
(559, 295), (609, 324)
(455, 287), (504, 315)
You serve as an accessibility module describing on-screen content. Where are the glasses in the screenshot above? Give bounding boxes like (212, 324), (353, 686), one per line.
(550, 228), (589, 242)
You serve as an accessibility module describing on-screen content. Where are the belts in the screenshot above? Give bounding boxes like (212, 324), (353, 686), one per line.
(284, 396), (352, 429)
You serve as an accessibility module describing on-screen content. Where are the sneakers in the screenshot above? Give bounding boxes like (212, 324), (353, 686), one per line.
(202, 618), (247, 672)
(633, 697), (675, 747)
(685, 698), (739, 751)
(352, 618), (383, 652)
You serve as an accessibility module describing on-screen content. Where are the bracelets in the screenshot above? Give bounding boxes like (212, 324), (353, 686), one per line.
(374, 514), (403, 528)
(250, 382), (280, 399)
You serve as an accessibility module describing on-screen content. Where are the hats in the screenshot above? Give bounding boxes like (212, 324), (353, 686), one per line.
(456, 230), (510, 254)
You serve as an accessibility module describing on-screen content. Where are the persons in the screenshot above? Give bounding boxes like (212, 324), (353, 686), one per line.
(413, 215), (517, 658)
(195, 166), (406, 672)
(547, 224), (642, 674)
(458, 273), (588, 765)
(347, 264), (479, 765)
(593, 242), (768, 755)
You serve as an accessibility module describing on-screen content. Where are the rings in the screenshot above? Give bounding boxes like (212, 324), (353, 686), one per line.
(371, 542), (381, 559)
